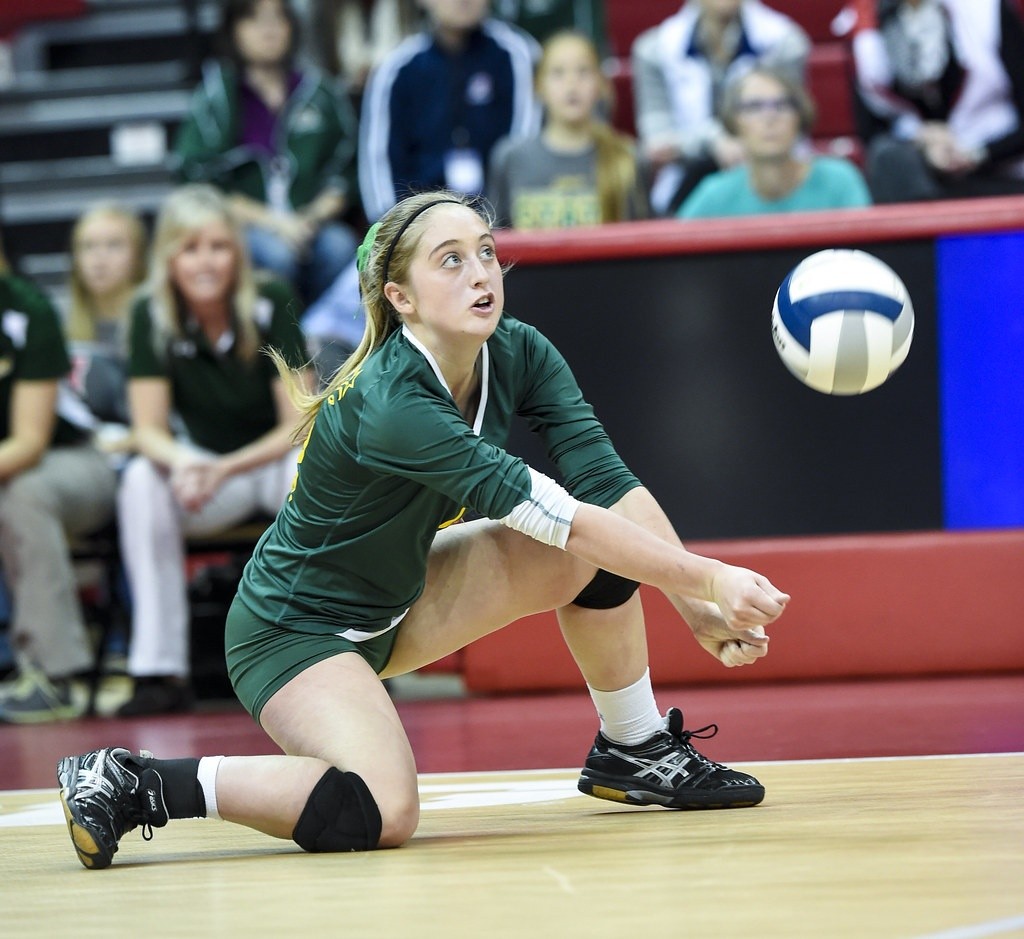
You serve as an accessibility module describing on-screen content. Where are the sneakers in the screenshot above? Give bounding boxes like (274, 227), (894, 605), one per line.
(58, 745), (170, 870)
(578, 707), (765, 807)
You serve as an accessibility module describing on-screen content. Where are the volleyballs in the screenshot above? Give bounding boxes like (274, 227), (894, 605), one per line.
(770, 249), (916, 397)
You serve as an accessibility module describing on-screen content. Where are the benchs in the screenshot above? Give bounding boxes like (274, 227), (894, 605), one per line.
(0, 0), (904, 646)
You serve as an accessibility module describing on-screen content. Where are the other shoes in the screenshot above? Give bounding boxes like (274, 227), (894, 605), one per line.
(1, 676), (89, 723)
(102, 674), (197, 716)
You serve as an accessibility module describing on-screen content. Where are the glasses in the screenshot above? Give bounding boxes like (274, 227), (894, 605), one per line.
(739, 98), (794, 112)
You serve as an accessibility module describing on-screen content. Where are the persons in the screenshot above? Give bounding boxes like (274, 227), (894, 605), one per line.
(0, 0), (1024, 729)
(55, 198), (791, 872)
